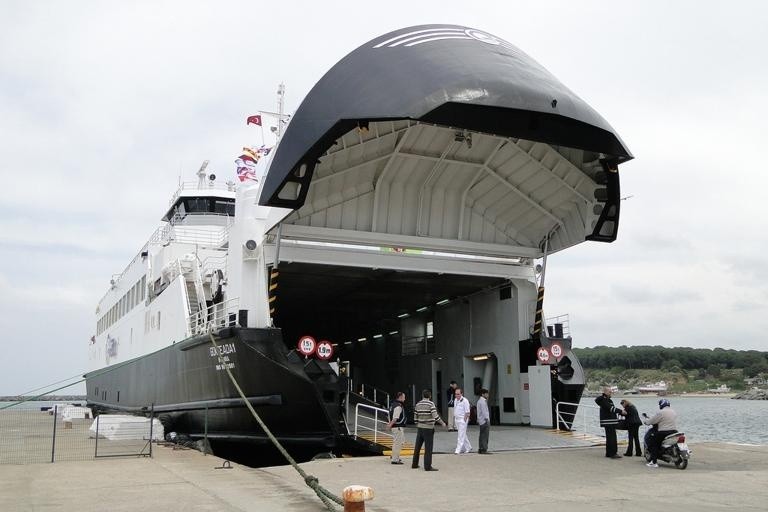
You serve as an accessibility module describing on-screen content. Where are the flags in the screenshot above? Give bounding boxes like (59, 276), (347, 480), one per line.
(235, 147), (269, 182)
(247, 115), (261, 126)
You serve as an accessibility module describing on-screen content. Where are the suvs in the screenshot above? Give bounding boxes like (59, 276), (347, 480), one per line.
(48, 404), (75, 416)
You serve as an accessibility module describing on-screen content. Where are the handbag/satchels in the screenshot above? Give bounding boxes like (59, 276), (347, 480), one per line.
(613, 412), (629, 429)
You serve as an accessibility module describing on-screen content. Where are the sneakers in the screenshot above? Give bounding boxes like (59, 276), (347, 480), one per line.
(604, 450), (642, 458)
(412, 465), (440, 471)
(391, 457), (405, 465)
(645, 459), (659, 468)
(453, 447), (492, 456)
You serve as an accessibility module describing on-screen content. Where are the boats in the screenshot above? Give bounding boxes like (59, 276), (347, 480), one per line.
(82, 22), (633, 454)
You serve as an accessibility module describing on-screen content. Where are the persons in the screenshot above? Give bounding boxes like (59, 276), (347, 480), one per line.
(644, 399), (677, 467)
(386, 392), (407, 464)
(595, 387), (627, 458)
(620, 399), (642, 456)
(447, 381), (458, 432)
(476, 389), (492, 454)
(453, 388), (472, 454)
(412, 389), (447, 471)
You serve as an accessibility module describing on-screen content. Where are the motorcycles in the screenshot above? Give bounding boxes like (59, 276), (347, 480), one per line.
(639, 412), (692, 472)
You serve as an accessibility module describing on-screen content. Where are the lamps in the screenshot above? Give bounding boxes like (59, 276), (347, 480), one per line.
(472, 354), (491, 361)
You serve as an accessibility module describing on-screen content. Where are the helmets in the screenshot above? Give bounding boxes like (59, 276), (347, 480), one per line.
(658, 398), (671, 410)
